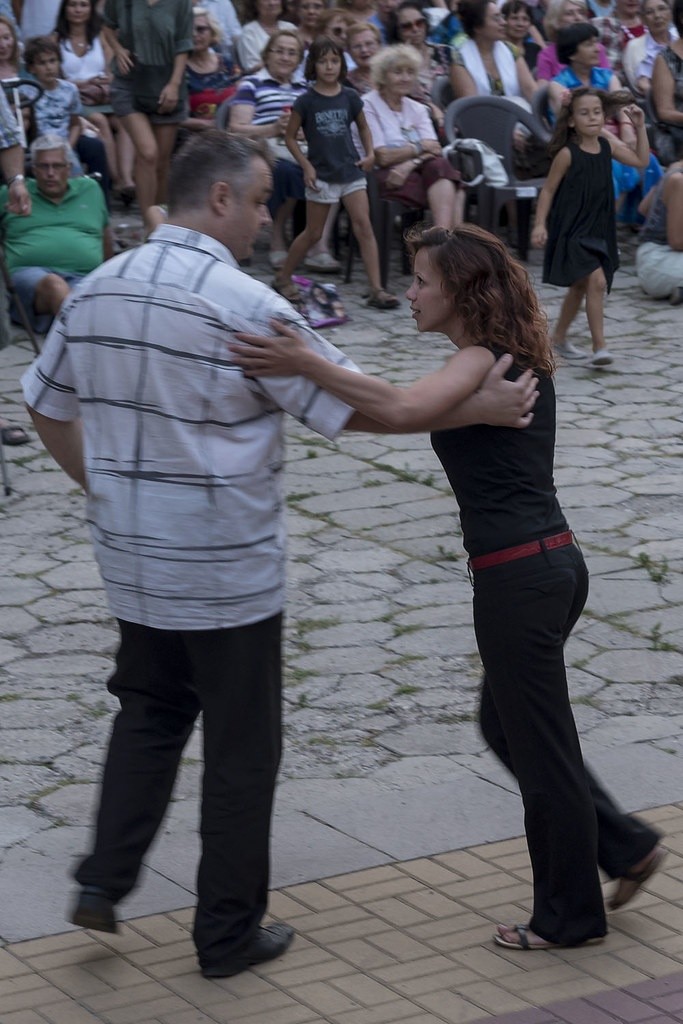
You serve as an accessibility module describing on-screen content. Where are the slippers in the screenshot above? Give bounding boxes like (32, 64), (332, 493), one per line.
(609, 847), (668, 911)
(493, 921), (603, 948)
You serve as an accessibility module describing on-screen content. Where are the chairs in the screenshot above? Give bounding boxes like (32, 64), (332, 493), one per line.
(344, 191), (426, 289)
(443, 92), (553, 262)
(217, 93), (234, 130)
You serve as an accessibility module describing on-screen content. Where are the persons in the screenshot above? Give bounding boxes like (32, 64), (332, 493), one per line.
(308, 284), (345, 318)
(229, 226), (673, 952)
(181, 0), (683, 308)
(526, 86), (650, 363)
(21, 129), (539, 979)
(0, 0), (197, 343)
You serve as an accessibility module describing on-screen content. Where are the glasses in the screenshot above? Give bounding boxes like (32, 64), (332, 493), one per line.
(324, 26), (347, 35)
(485, 13), (505, 21)
(399, 17), (427, 31)
(33, 162), (69, 171)
(267, 48), (302, 57)
(193, 23), (211, 34)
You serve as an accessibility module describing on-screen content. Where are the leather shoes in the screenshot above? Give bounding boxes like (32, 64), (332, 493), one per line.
(68, 888), (117, 935)
(200, 922), (294, 980)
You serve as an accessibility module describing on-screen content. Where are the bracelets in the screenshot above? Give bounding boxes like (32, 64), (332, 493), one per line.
(409, 141), (423, 156)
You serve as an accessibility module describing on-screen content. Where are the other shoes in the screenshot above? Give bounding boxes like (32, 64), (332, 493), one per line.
(549, 340), (585, 359)
(591, 349), (613, 365)
(269, 248), (289, 266)
(670, 287), (683, 304)
(304, 248), (342, 271)
(111, 184), (137, 206)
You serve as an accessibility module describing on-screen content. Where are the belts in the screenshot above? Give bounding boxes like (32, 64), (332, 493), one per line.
(469, 530), (573, 569)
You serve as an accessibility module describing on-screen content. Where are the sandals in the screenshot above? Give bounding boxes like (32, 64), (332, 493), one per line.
(366, 288), (400, 309)
(271, 272), (302, 303)
(0, 418), (31, 445)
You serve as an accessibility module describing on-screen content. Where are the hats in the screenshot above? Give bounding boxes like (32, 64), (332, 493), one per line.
(604, 90), (636, 118)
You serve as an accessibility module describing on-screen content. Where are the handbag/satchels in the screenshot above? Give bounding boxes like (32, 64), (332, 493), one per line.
(79, 80), (113, 106)
(187, 86), (236, 119)
(443, 137), (509, 188)
